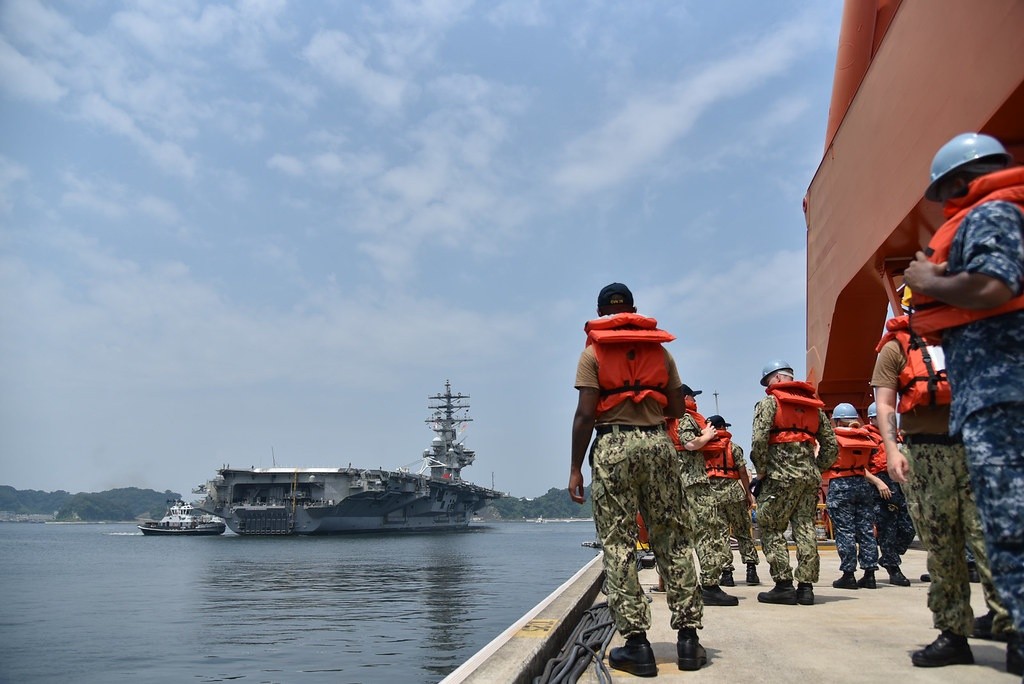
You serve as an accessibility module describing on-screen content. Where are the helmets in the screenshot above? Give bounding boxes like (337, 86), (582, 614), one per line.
(831, 403), (859, 419)
(901, 284), (912, 307)
(867, 402), (877, 417)
(760, 360), (794, 386)
(925, 132), (1014, 203)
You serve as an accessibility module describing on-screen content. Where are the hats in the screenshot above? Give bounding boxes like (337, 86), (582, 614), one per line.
(704, 415), (731, 428)
(682, 384), (702, 398)
(598, 282), (633, 306)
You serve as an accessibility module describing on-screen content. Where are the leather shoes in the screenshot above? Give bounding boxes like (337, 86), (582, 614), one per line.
(858, 570), (876, 589)
(833, 572), (858, 589)
(677, 631), (708, 671)
(702, 584), (739, 606)
(796, 583), (815, 605)
(758, 581), (797, 605)
(609, 640), (657, 677)
(968, 609), (996, 639)
(746, 565), (759, 584)
(912, 630), (975, 667)
(1006, 635), (1024, 675)
(718, 572), (734, 587)
(890, 568), (910, 586)
(878, 556), (884, 568)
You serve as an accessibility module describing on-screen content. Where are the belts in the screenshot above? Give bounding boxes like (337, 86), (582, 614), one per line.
(597, 424), (664, 436)
(903, 433), (962, 446)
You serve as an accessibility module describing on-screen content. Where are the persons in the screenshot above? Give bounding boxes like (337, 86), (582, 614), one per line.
(750, 360), (838, 605)
(822, 403), (880, 589)
(702, 416), (760, 586)
(863, 402), (916, 586)
(903, 131), (1024, 637)
(569, 282), (706, 676)
(870, 285), (1024, 676)
(665, 384), (738, 605)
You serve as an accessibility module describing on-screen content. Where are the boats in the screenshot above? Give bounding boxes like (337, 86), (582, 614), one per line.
(188, 378), (506, 539)
(137, 495), (227, 537)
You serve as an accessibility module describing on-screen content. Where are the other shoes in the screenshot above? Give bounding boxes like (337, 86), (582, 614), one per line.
(968, 567), (981, 583)
(921, 575), (931, 583)
